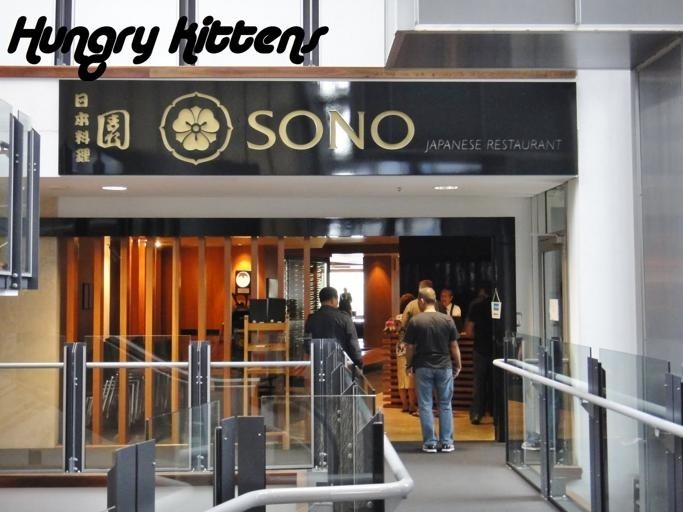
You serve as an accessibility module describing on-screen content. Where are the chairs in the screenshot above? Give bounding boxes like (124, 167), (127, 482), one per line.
(79, 334), (182, 428)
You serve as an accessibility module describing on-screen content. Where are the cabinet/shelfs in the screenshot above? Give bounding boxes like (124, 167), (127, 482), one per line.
(239, 313), (291, 450)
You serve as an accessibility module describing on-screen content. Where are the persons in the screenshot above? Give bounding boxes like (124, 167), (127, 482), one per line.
(392, 276), (515, 456)
(339, 288), (354, 310)
(304, 286), (369, 460)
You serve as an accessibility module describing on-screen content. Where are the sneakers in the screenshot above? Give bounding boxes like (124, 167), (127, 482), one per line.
(422, 444), (437, 453)
(442, 444), (455, 452)
